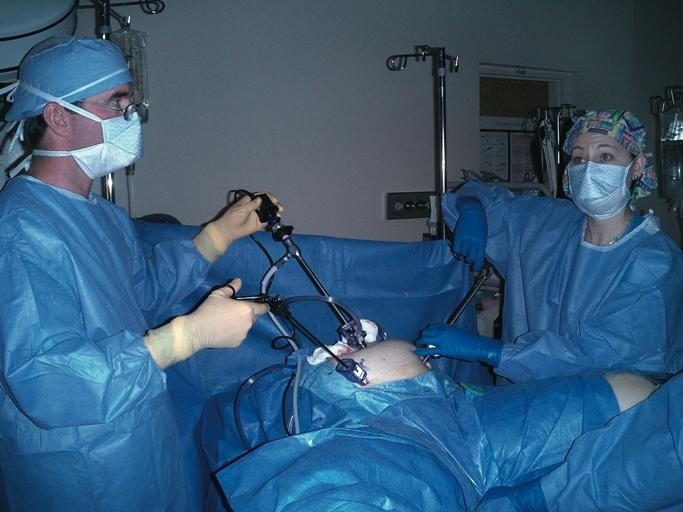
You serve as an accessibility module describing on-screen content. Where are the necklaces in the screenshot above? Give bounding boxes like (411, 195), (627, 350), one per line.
(607, 220), (631, 245)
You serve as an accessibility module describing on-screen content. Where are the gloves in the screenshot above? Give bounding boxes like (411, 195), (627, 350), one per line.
(412, 322), (503, 368)
(450, 195), (488, 274)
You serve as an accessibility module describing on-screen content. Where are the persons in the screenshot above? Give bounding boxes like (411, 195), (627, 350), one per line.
(324, 338), (658, 490)
(412, 105), (683, 382)
(0, 34), (284, 511)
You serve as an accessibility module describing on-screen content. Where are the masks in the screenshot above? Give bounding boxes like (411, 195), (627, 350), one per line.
(48, 97), (143, 181)
(565, 157), (636, 223)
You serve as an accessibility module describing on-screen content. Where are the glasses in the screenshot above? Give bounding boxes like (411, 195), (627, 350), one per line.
(79, 99), (148, 123)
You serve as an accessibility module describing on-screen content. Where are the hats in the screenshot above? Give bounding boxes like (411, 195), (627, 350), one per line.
(561, 109), (658, 206)
(3, 35), (133, 122)
